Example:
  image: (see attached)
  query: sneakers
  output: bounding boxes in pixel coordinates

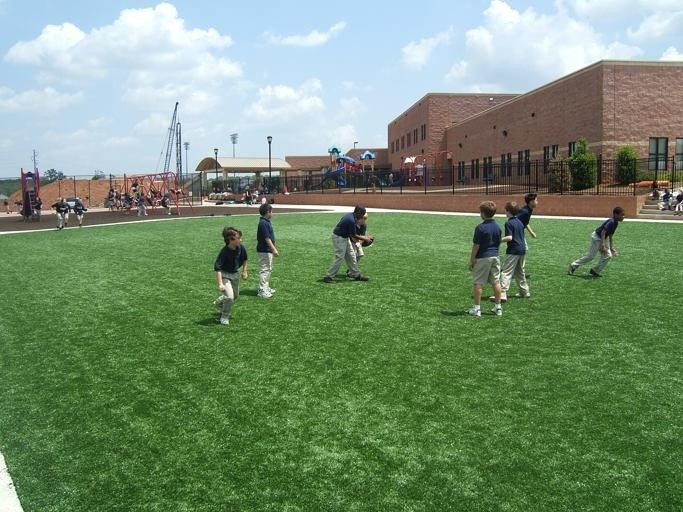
[256,292,273,299]
[567,262,577,275]
[356,274,369,281]
[323,276,334,283]
[220,318,230,325]
[523,273,531,278]
[268,287,276,293]
[213,301,222,308]
[346,269,355,278]
[466,309,481,316]
[589,269,602,278]
[491,308,502,316]
[489,293,507,302]
[515,293,530,298]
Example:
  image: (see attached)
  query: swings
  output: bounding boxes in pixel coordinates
[109,172,182,209]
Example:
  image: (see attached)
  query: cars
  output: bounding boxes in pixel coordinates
[63,198,79,210]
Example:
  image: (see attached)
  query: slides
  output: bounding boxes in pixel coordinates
[19,191,33,216]
[329,166,347,186]
[375,176,389,186]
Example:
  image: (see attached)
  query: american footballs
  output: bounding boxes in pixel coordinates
[362,235,374,246]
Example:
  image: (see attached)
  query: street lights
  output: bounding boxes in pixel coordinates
[229,133,239,159]
[213,148,219,182]
[267,135,273,194]
[183,141,190,180]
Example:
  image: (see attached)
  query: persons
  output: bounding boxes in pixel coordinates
[3,196,87,230]
[651,180,683,216]
[467,201,503,316]
[240,186,259,206]
[488,201,530,303]
[323,202,373,284]
[213,226,248,325]
[110,179,182,216]
[515,194,538,238]
[568,207,625,277]
[256,204,279,298]
[346,211,368,278]
